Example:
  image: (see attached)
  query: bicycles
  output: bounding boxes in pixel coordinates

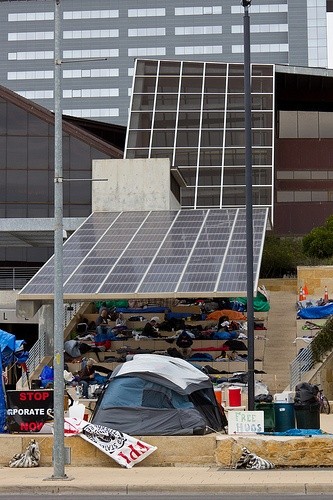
[315,384,331,415]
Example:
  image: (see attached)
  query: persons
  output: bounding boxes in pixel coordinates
[96,310,109,327]
[115,311,127,326]
[76,360,96,399]
[176,330,193,348]
[26,436,48,459]
[142,320,161,338]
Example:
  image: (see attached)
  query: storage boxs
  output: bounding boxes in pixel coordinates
[274,392,294,404]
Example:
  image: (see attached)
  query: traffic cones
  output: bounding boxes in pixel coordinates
[323,285,330,301]
[298,286,304,301]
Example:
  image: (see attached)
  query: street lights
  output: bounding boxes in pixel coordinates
[237,0,255,410]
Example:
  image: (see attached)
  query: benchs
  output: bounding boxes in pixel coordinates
[65,290,270,402]
[295,318,329,358]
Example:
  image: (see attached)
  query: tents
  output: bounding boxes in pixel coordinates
[88,353,228,435]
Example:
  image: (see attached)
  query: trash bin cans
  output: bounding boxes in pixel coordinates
[274,403,295,432]
[293,403,321,429]
[254,402,276,432]
